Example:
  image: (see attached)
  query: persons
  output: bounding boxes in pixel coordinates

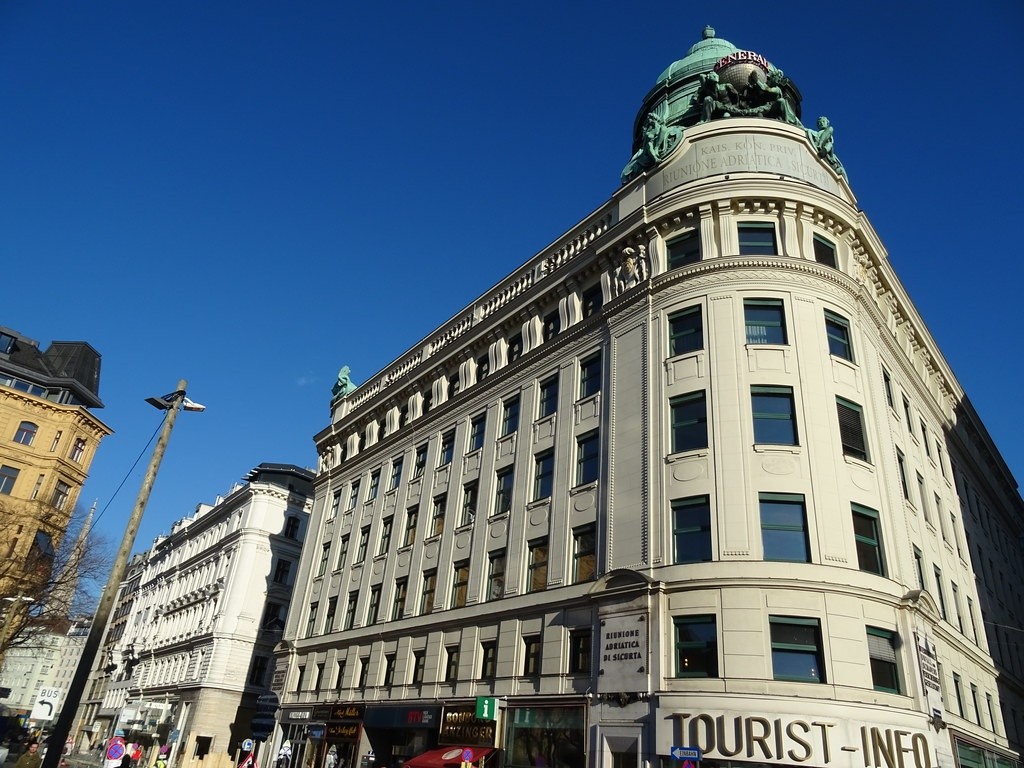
[808,116,834,158]
[113,742,143,768]
[14,741,43,768]
[691,71,739,127]
[750,69,801,124]
[642,112,667,164]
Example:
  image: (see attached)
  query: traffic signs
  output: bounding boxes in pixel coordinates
[671,747,700,761]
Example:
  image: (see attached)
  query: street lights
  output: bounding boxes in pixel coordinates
[39,379,206,768]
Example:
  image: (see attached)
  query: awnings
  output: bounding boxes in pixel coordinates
[398,745,500,768]
